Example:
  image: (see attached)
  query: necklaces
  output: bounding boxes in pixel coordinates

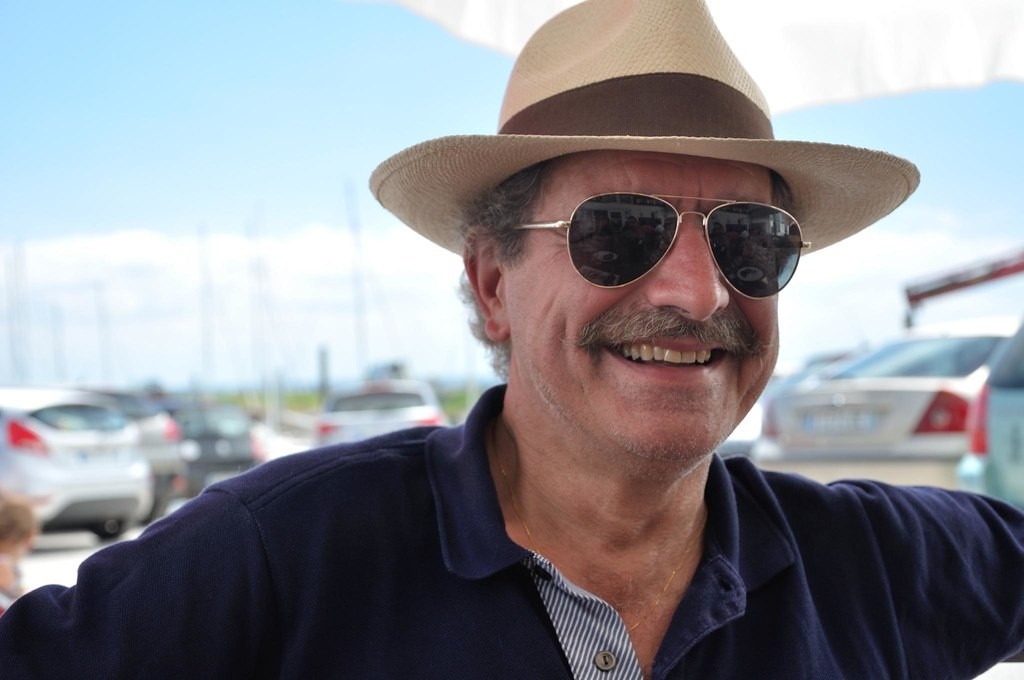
[491,416,708,631]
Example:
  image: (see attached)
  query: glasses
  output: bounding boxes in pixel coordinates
[511,190,812,300]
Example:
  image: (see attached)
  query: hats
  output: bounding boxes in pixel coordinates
[366,0,922,267]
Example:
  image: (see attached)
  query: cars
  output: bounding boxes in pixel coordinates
[749,317,1024,505]
[0,386,154,540]
[163,389,268,499]
[309,377,450,449]
[97,386,186,531]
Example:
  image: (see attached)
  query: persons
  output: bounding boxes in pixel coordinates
[591,208,802,295]
[0,0,1024,680]
[0,495,41,618]
